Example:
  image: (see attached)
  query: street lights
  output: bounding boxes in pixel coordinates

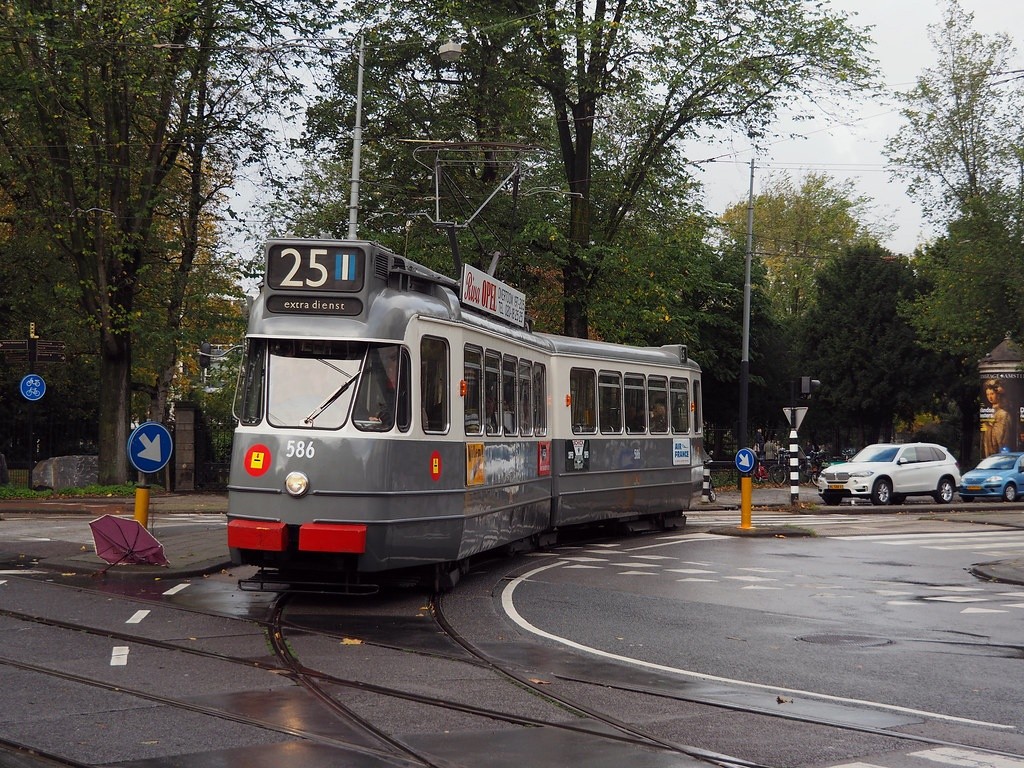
[790,378,821,505]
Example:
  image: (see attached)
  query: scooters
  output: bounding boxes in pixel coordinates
[704,451,717,502]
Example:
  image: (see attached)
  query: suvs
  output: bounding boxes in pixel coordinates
[817,443,963,505]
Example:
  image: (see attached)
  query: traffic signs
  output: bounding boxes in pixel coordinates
[0,339,66,363]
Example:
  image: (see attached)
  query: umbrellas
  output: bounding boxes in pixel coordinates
[88,514,171,574]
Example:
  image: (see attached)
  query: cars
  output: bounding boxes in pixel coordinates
[958,451,1024,503]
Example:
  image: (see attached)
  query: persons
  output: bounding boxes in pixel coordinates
[626,407,644,433]
[650,398,667,432]
[369,405,428,430]
[754,429,829,480]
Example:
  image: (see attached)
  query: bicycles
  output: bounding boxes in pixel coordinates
[749,446,855,487]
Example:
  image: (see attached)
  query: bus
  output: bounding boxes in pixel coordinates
[197,237,704,604]
